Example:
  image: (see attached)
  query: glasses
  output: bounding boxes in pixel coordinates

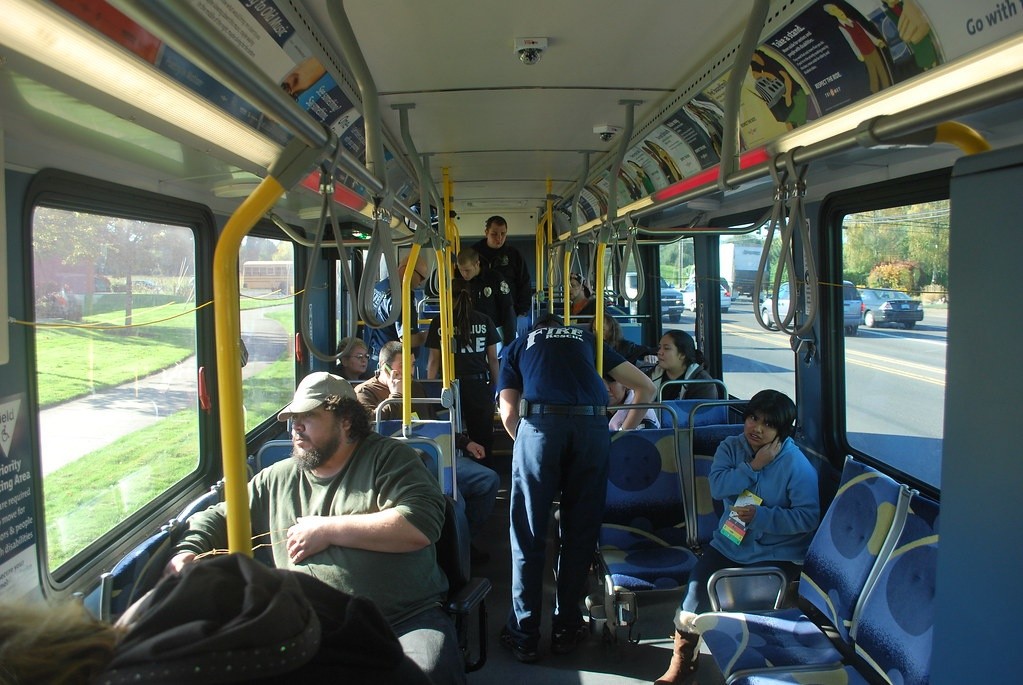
[414,269,426,282]
[344,353,370,360]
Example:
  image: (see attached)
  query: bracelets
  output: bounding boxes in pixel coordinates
[617,424,624,431]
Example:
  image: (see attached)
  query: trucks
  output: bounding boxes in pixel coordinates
[688,242,772,302]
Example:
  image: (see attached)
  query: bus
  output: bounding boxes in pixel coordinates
[242,261,294,295]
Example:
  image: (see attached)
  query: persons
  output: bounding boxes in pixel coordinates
[471,216,532,317]
[423,277,503,468]
[161,371,467,685]
[654,389,821,685]
[452,247,516,348]
[354,341,499,568]
[333,337,373,380]
[570,272,612,324]
[588,313,658,370]
[0,552,427,685]
[363,253,429,379]
[500,320,659,663]
[602,367,661,431]
[643,329,719,399]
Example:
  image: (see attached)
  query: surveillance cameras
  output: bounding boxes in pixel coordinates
[514,37,548,65]
[593,126,622,143]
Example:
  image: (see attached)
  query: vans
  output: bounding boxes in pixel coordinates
[62,273,112,294]
[758,279,864,336]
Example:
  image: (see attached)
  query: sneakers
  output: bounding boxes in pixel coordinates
[550,623,591,653]
[497,623,540,664]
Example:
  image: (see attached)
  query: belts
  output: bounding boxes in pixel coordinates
[528,405,607,416]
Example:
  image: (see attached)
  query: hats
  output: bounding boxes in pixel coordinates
[277,371,358,423]
[570,272,592,299]
[532,314,563,328]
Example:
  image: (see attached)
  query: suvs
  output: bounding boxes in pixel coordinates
[602,271,685,322]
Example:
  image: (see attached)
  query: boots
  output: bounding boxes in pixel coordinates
[653,608,703,685]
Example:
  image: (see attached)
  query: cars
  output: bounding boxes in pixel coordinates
[115,280,156,293]
[685,274,731,294]
[856,288,925,329]
[679,280,731,314]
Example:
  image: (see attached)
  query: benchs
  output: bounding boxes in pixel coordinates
[98,298,940,684]
